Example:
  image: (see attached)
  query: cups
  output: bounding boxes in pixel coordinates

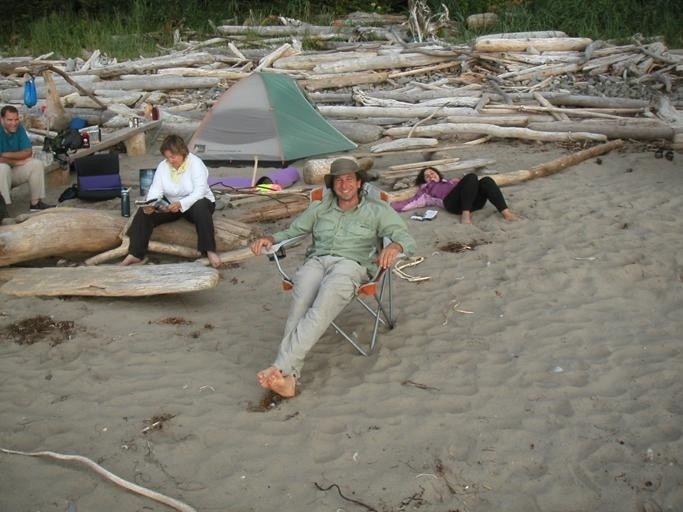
[134,118,138,128]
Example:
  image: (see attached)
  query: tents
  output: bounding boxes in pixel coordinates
[185,70,359,166]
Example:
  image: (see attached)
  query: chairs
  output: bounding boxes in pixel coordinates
[259,180,395,358]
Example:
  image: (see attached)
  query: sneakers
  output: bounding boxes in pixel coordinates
[30,200,55,212]
[25,80,37,107]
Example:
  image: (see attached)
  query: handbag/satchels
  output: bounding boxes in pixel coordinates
[43,127,82,153]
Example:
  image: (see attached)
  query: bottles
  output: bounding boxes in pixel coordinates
[128,114,133,128]
[152,105,158,121]
[121,187,130,217]
[81,132,90,147]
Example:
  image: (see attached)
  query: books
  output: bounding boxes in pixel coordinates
[134,198,172,214]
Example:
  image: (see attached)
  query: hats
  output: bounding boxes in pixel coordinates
[325,158,366,187]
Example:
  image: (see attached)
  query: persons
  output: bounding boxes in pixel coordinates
[391,167,517,224]
[122,135,220,269]
[0,105,57,218]
[251,158,417,399]
